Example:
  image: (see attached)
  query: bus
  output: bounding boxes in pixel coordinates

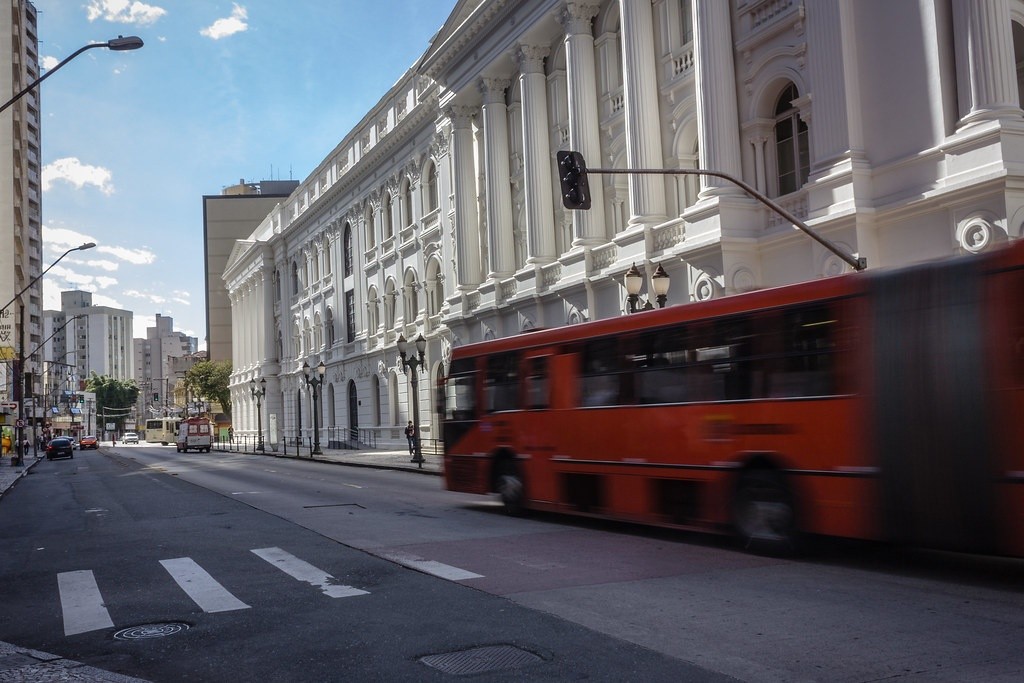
[439,235,1024,579]
[174,417,213,453]
[145,416,184,445]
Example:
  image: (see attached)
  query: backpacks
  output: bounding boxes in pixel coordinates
[406,425,414,436]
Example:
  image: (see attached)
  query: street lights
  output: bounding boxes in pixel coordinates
[623,259,671,364]
[396,332,428,463]
[249,377,267,451]
[14,305,89,466]
[32,348,81,458]
[303,360,326,454]
[153,378,169,417]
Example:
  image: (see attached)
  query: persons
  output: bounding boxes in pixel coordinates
[23,438,31,455]
[227,425,234,442]
[36,436,41,450]
[405,421,415,455]
[14,439,19,453]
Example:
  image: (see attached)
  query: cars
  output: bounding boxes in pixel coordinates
[123,433,139,444]
[79,435,99,450]
[45,436,77,460]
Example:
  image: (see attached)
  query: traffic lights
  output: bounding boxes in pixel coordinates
[80,394,85,404]
[154,392,159,401]
[557,150,594,210]
